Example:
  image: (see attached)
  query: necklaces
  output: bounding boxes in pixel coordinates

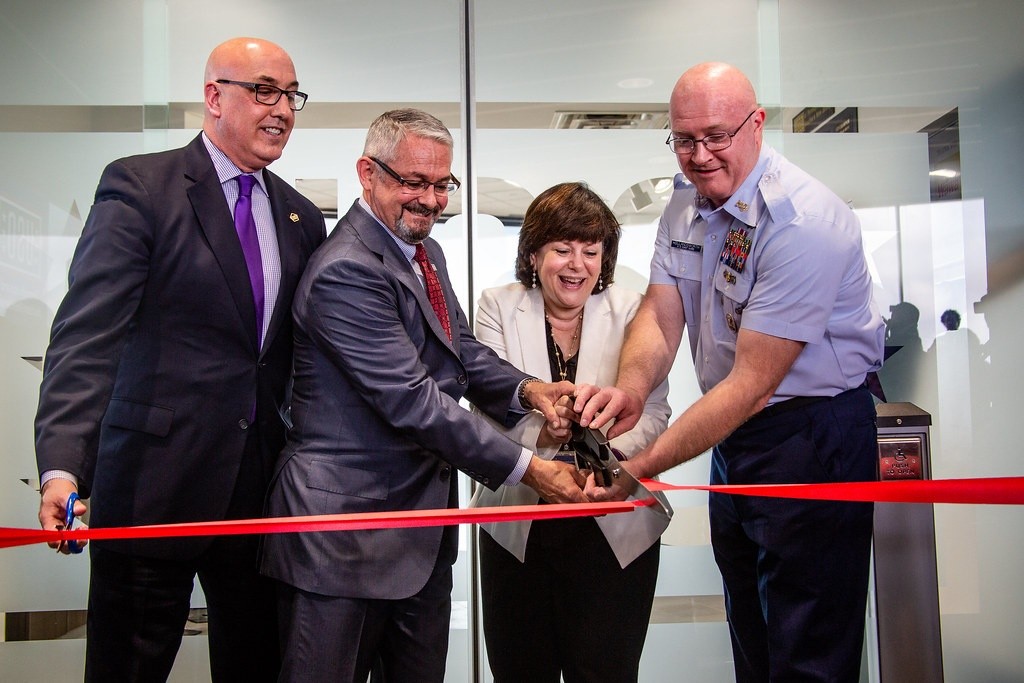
[543,305,582,382]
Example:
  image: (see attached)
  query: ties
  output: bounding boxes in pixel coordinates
[412,242,453,347]
[230,174,265,350]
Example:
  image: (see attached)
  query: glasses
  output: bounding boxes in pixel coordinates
[369,156,461,197]
[216,79,309,111]
[665,110,755,155]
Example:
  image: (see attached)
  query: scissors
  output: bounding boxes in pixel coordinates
[54,491,84,554]
[567,396,671,522]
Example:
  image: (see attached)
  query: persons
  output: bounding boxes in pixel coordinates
[940,310,961,329]
[881,302,922,352]
[465,182,671,683]
[574,63,885,683]
[252,108,603,683]
[34,37,326,683]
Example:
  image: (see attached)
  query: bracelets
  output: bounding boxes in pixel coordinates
[519,378,545,409]
